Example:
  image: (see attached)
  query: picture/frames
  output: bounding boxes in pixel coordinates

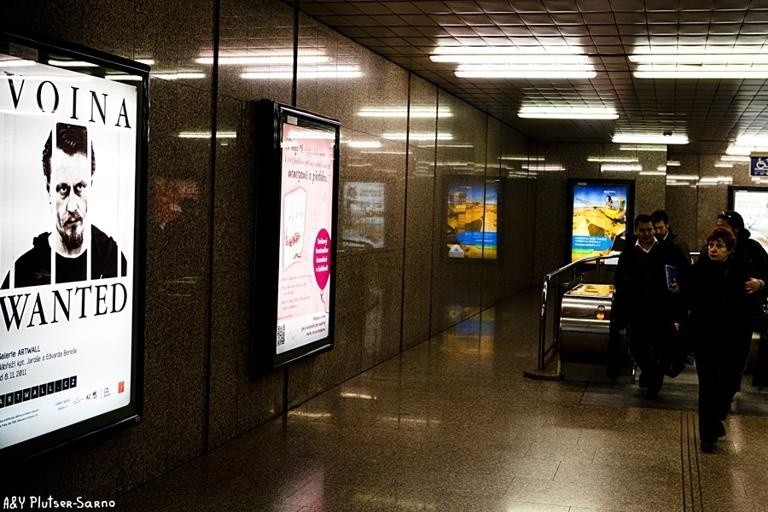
[1,18,157,474]
[437,172,509,270]
[556,176,636,265]
[243,95,342,392]
[340,174,398,267]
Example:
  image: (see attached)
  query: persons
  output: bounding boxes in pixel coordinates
[610,210,767,453]
[1,123,127,289]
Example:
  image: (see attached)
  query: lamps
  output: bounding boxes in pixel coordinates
[421,51,768,146]
